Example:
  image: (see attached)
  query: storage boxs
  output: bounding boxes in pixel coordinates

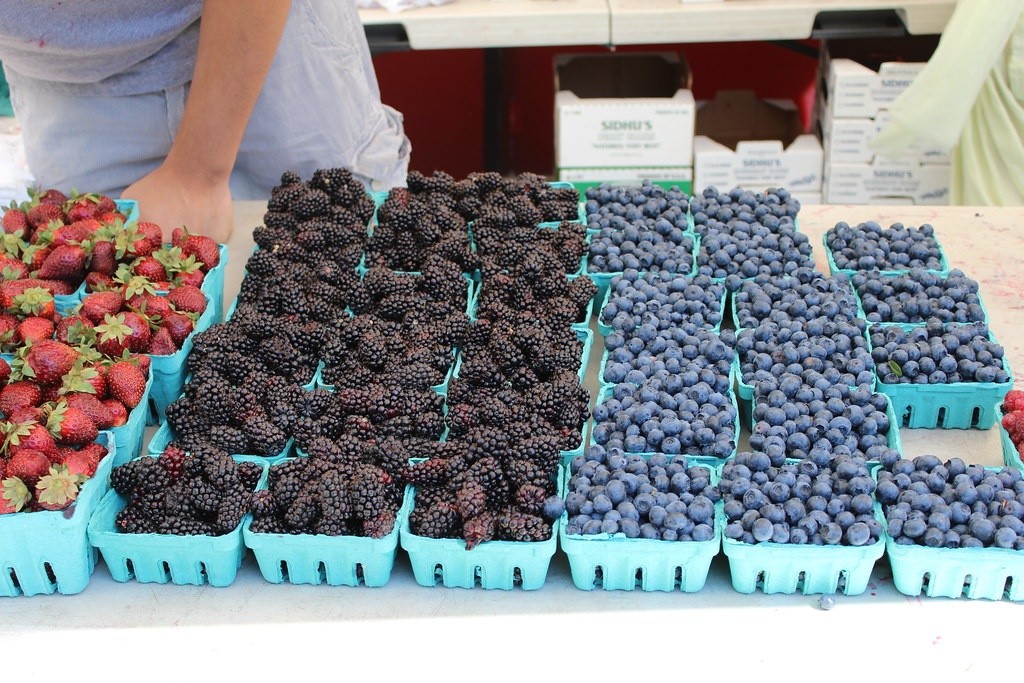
[554,38,966,202]
[0,168,1024,600]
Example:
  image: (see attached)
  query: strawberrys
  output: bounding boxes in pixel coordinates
[1001,389,1024,464]
[0,183,219,515]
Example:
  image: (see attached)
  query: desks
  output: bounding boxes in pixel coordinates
[358,0,960,182]
[0,197,1024,630]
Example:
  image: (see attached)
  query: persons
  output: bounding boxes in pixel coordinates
[0,0,411,245]
